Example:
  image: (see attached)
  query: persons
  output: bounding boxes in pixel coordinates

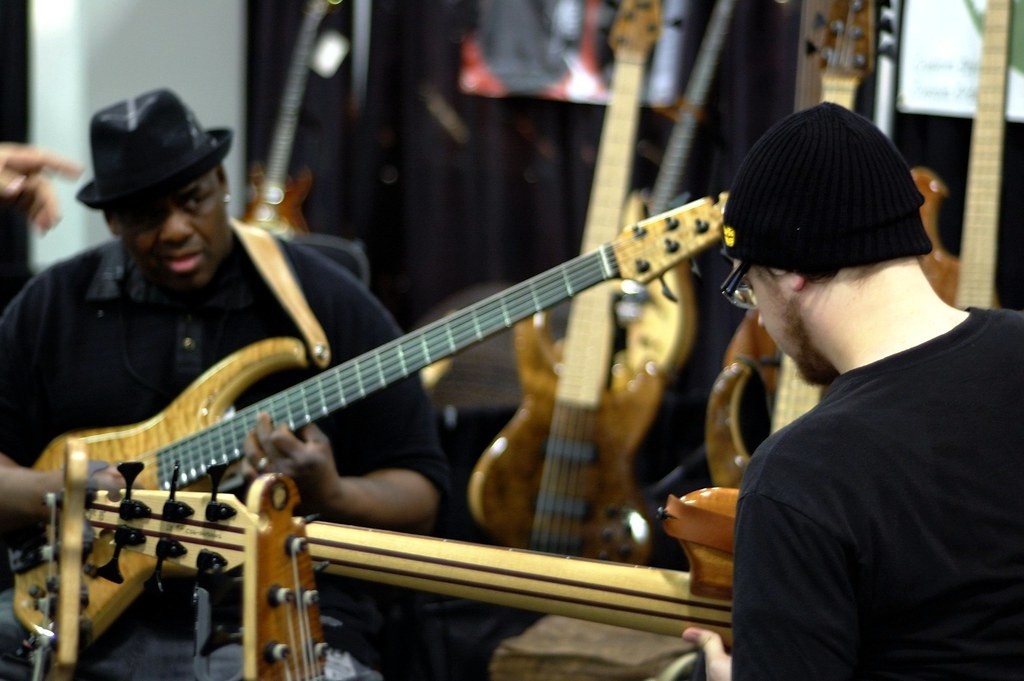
[681,102,1023,681]
[0,86,533,680]
[0,141,83,235]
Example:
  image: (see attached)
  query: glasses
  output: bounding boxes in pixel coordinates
[719,260,756,310]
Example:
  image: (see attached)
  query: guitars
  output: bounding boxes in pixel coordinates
[36,185,732,495]
[704,0,1024,488]
[236,0,342,236]
[466,1,700,568]
[40,437,735,681]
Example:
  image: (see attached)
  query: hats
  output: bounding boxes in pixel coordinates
[720,102,932,273]
[75,89,237,209]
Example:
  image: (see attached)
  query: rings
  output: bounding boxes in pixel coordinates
[256,454,269,470]
